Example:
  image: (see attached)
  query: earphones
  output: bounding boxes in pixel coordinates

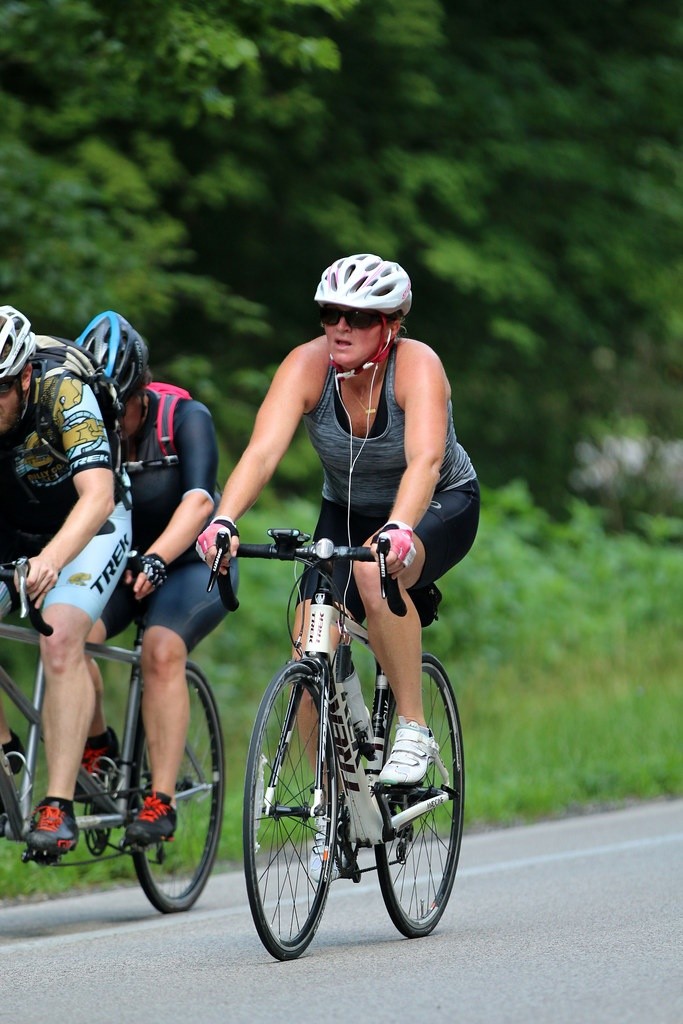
[387,329,392,343]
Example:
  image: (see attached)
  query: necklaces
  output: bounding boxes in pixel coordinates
[344,381,376,414]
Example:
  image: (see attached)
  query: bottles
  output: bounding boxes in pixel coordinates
[342,661,368,733]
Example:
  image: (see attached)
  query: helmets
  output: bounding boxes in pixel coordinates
[0,304,36,380]
[74,310,147,402]
[314,253,412,316]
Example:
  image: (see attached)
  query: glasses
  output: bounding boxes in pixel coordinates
[0,358,30,393]
[318,304,380,329]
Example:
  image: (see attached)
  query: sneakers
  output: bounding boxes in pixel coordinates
[125,789,177,843]
[74,725,121,798]
[27,800,78,853]
[378,716,439,786]
[309,813,355,882]
[2,728,24,775]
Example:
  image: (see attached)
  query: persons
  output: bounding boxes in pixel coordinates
[196,253,481,882]
[76,312,241,845]
[0,304,132,855]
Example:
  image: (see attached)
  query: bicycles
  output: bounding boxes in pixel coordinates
[0,547,228,914]
[209,529,469,963]
